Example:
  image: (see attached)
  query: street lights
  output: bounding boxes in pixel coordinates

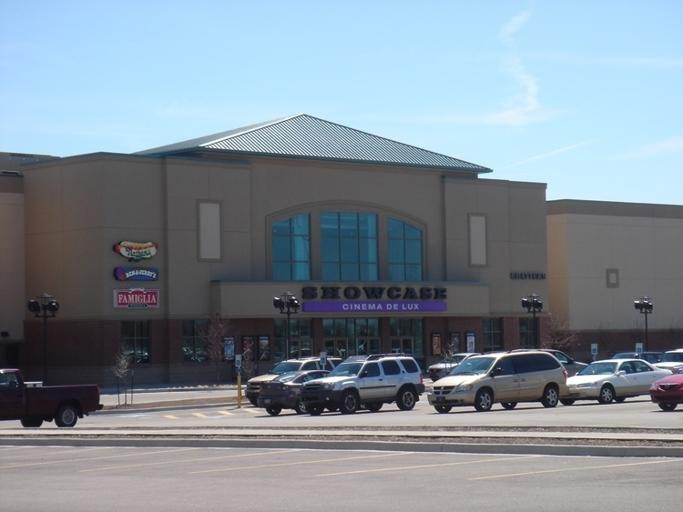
[520,294,544,348]
[273,290,300,360]
[27,292,60,385]
[632,295,653,351]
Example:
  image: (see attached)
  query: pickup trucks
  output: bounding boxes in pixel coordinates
[0,368,104,427]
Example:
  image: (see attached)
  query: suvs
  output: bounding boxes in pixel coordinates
[428,352,490,383]
[299,353,426,416]
[511,348,595,377]
[427,350,570,414]
[244,356,350,407]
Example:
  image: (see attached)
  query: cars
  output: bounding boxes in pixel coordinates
[256,370,336,415]
[558,359,673,406]
[605,348,683,374]
[649,372,683,411]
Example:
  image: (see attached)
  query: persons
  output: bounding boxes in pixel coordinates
[445,343,455,358]
